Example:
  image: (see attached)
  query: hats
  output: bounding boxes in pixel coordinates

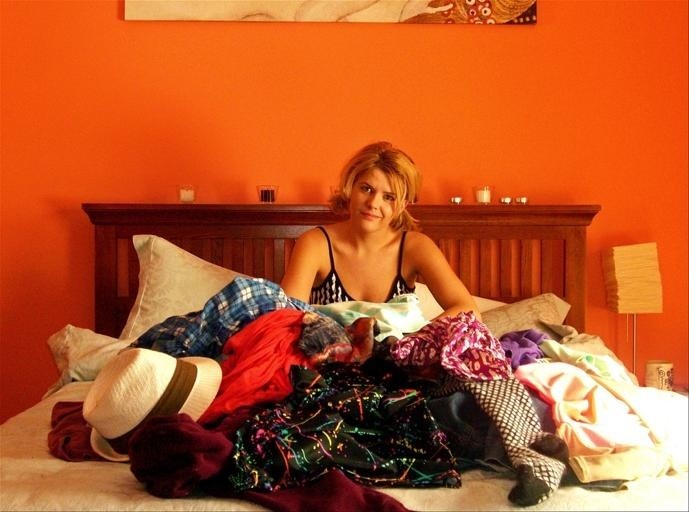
[81,346,223,463]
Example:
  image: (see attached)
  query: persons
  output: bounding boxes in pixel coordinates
[280,140,484,328]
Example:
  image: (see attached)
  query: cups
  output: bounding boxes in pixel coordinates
[176,184,199,203]
[256,185,279,204]
[472,184,495,205]
[644,360,674,392]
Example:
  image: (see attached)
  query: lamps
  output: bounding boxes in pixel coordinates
[600,241,666,375]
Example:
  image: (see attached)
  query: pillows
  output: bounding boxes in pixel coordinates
[48,231,281,391]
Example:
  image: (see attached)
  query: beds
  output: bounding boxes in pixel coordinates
[0,202,687,511]
